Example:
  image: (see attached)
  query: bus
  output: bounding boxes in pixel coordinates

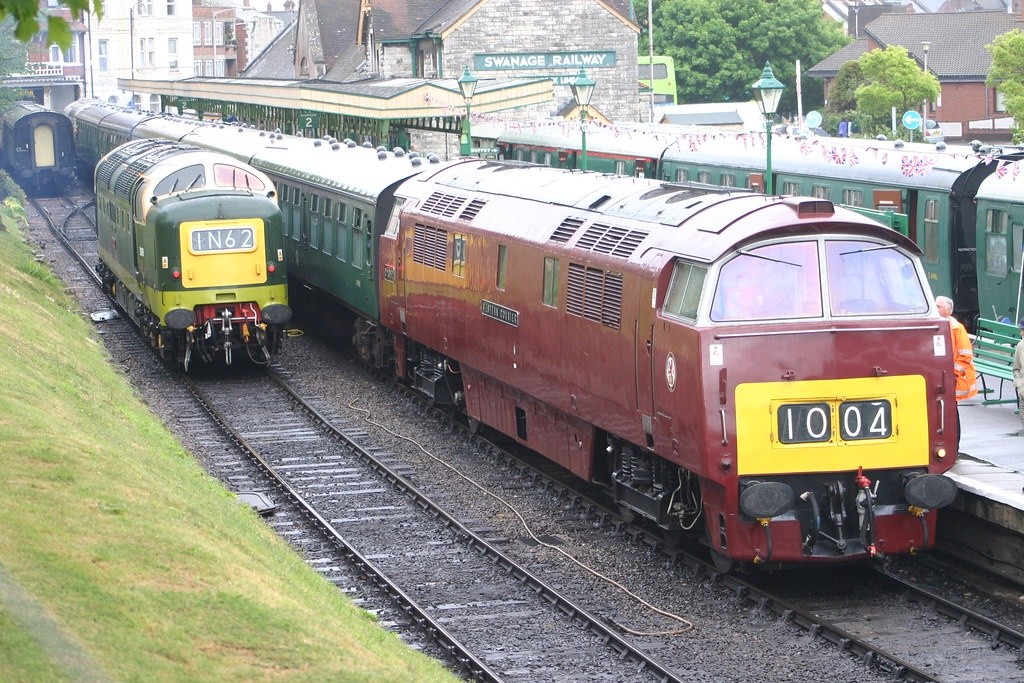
[638,56,679,106]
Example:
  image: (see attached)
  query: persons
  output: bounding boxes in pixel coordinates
[839,118,847,137]
[1013,317,1024,426]
[935,296,977,462]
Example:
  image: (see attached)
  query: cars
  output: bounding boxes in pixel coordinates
[925,119,945,145]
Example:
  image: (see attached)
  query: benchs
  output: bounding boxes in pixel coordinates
[971,318,1023,415]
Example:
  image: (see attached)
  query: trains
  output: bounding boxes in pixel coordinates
[0,98,82,196]
[61,99,961,588]
[91,136,295,368]
[496,115,1023,336]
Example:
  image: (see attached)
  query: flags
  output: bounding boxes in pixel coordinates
[424,94,1021,180]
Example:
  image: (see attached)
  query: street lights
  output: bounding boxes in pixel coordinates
[750,60,786,195]
[920,40,932,138]
[567,66,595,171]
[457,66,478,161]
